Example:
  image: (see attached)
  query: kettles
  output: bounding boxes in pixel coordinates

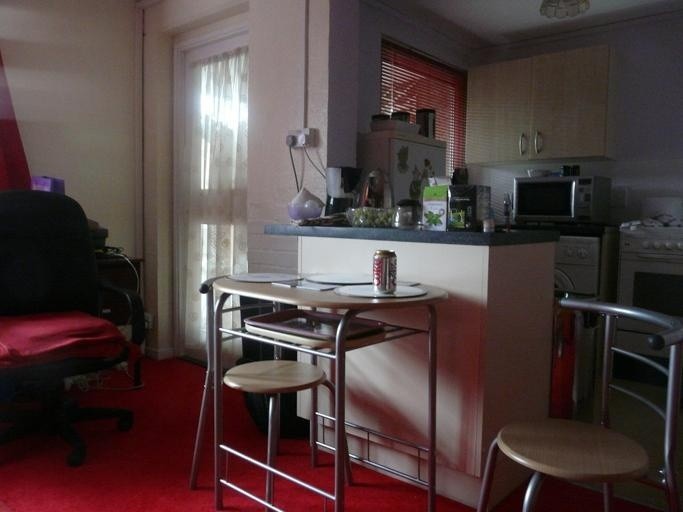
[392,198,421,231]
[353,164,391,208]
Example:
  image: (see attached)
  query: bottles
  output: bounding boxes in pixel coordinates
[559,166,579,175]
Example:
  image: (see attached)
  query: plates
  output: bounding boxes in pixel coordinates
[225,269,302,284]
[306,272,378,284]
[334,283,428,299]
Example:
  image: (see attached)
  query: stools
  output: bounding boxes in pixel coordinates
[217,355,355,512]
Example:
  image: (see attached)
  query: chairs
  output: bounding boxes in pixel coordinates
[1,190,144,469]
[475,296,683,511]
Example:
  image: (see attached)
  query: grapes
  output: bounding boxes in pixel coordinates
[352,207,395,226]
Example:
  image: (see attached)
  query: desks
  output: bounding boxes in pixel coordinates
[209,273,449,511]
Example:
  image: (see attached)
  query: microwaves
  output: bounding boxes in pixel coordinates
[512,176,595,223]
[448,184,481,225]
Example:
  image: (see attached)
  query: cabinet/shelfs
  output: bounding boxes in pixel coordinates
[463,45,623,169]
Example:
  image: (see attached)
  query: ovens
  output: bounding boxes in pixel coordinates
[618,252,683,333]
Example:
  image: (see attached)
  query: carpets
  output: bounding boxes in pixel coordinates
[1,357,661,512]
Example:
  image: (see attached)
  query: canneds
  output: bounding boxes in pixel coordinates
[374,250,397,294]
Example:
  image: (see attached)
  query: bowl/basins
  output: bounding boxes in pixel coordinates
[343,206,391,229]
[526,167,550,177]
[370,120,420,137]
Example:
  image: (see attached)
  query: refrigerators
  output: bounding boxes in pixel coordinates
[354,133,447,216]
